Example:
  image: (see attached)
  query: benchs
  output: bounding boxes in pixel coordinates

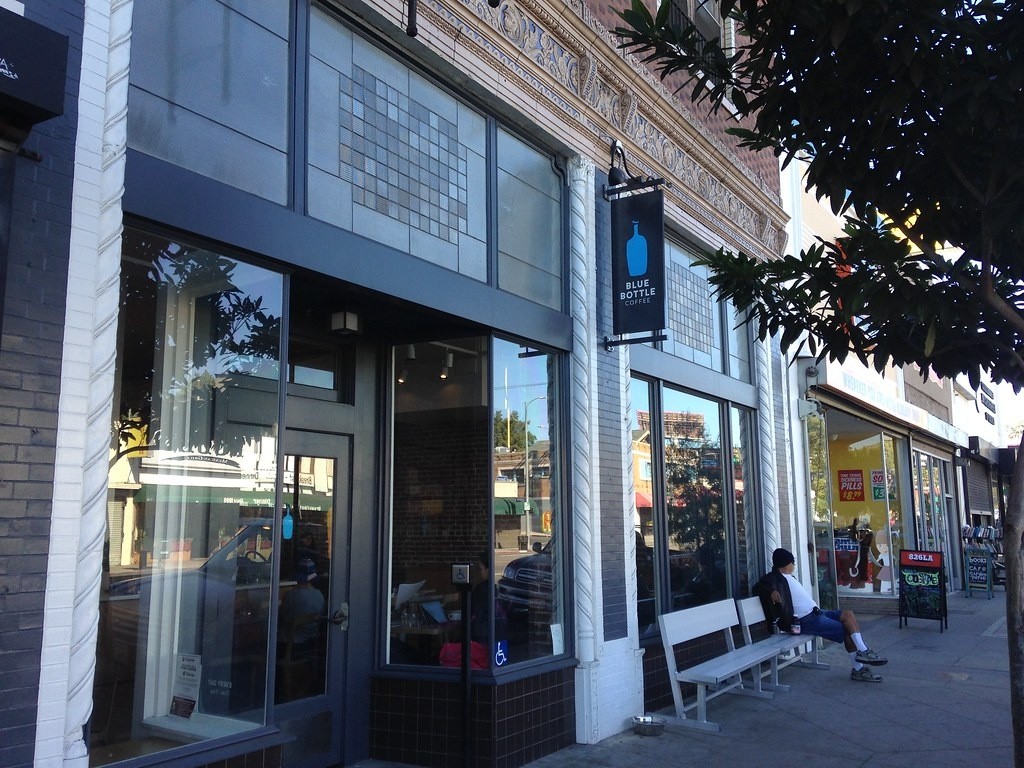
[728,595,830,693]
[645,599,781,730]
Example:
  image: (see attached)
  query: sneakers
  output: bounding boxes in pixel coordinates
[855,648,889,666]
[851,665,884,683]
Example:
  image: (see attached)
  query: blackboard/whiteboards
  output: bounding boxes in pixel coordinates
[966,548,993,589]
[898,549,948,620]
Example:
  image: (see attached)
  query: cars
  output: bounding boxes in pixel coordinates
[104,521,333,669]
[499,532,646,625]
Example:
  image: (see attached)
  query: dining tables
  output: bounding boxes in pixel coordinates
[390,600,462,665]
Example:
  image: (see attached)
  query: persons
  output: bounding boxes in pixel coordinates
[299,530,317,551]
[749,546,888,683]
[464,547,532,649]
[682,547,729,608]
[259,559,329,647]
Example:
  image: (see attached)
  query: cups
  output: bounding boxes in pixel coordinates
[448,611,462,622]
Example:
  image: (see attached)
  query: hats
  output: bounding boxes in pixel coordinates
[772,548,794,569]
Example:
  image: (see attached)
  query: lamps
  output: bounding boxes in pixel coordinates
[608,145,641,186]
[397,369,409,384]
[434,352,455,387]
[330,311,359,337]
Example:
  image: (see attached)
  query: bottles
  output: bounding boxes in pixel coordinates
[791,614,801,636]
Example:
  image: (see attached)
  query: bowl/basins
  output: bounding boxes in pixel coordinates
[632,716,667,736]
